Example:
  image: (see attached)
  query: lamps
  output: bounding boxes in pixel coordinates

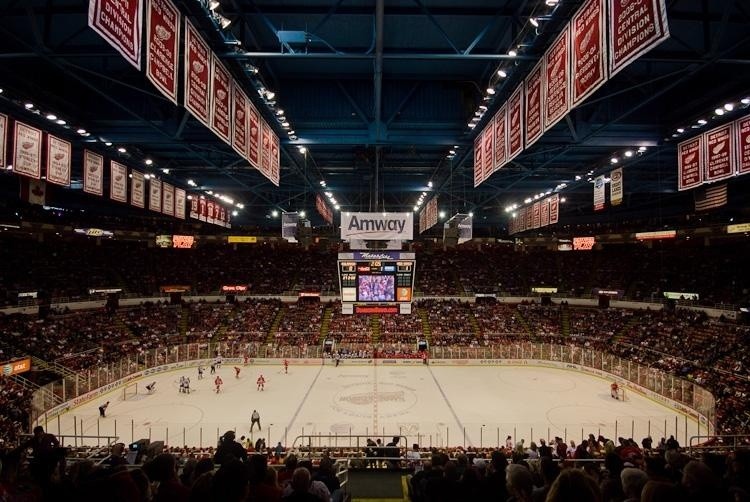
[206,0,232,30]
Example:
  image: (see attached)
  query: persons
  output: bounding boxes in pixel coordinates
[0,241,750,502]
